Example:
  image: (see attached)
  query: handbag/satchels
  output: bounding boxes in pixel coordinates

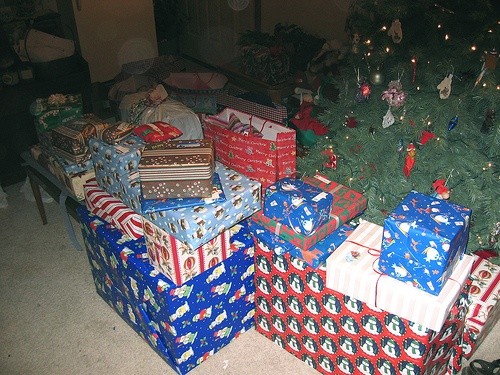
[119,89,204,141]
[190,106,298,200]
[21,17,77,63]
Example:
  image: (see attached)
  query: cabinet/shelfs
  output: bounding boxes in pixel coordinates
[0,52,92,189]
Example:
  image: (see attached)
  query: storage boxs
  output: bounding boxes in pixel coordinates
[28,43,500,375]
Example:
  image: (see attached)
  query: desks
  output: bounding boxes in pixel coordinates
[235,68,295,104]
[27,164,79,225]
